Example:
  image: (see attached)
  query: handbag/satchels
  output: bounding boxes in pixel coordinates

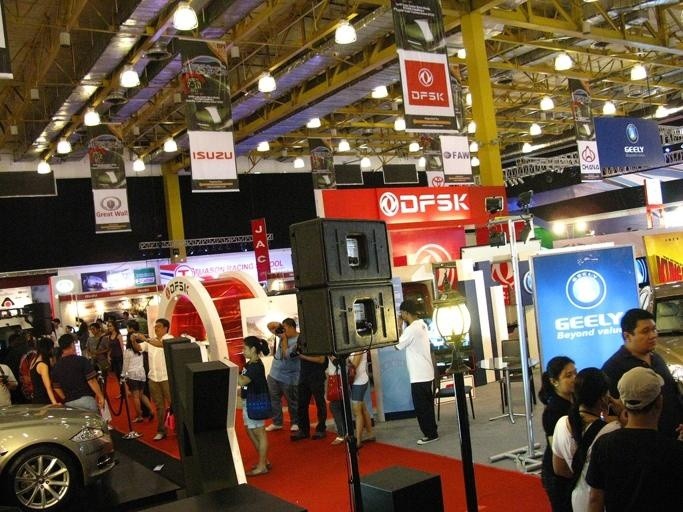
[327,375,342,401]
[246,391,273,419]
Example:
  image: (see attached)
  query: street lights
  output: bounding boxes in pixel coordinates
[431,275,479,507]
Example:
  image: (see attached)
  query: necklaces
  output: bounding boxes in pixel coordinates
[578,410,601,418]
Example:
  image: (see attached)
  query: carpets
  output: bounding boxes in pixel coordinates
[99,368,552,512]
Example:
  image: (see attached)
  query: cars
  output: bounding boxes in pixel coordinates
[0,403,119,507]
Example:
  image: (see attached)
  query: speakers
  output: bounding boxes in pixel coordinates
[288,218,392,289]
[358,464,445,511]
[295,284,398,356]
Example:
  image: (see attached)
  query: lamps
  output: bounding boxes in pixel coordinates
[486,190,535,249]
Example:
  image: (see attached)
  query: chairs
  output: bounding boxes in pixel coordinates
[430,353,475,421]
[498,339,536,416]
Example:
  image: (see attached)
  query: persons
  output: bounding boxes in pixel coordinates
[552,362,627,512]
[535,354,580,512]
[585,366,683,512]
[598,308,683,441]
[0,297,381,483]
[393,300,440,444]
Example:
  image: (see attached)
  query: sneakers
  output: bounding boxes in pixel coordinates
[265,424,283,431]
[290,432,310,438]
[153,433,167,440]
[132,417,144,423]
[417,435,439,444]
[290,424,299,431]
[348,367,356,383]
[312,430,326,439]
[361,436,376,441]
[332,434,348,445]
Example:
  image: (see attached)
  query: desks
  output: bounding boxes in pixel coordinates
[473,354,540,423]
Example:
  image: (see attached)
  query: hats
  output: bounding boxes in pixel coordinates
[400,300,421,316]
[616,367,665,410]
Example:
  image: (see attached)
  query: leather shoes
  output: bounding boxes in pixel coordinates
[246,468,267,476]
[252,463,273,469]
